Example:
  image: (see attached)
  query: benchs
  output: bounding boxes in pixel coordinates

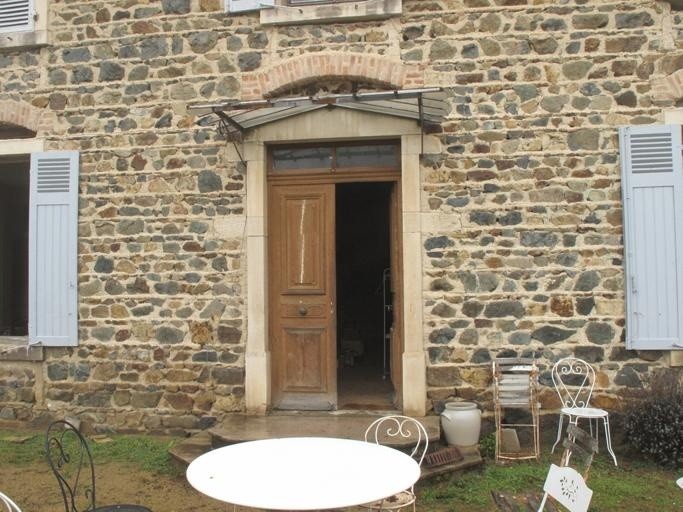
[490,422,599,512]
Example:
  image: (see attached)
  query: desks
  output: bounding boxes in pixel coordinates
[185,437,421,512]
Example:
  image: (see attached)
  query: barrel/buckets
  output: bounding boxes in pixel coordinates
[441,402,482,447]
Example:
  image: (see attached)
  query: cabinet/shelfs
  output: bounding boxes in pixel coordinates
[381,264,392,380]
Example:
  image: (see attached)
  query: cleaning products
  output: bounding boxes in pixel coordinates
[344,344,355,368]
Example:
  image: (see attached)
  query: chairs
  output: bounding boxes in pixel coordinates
[537,463,594,512]
[551,357,618,467]
[358,416,429,512]
[45,419,153,512]
[491,357,541,465]
